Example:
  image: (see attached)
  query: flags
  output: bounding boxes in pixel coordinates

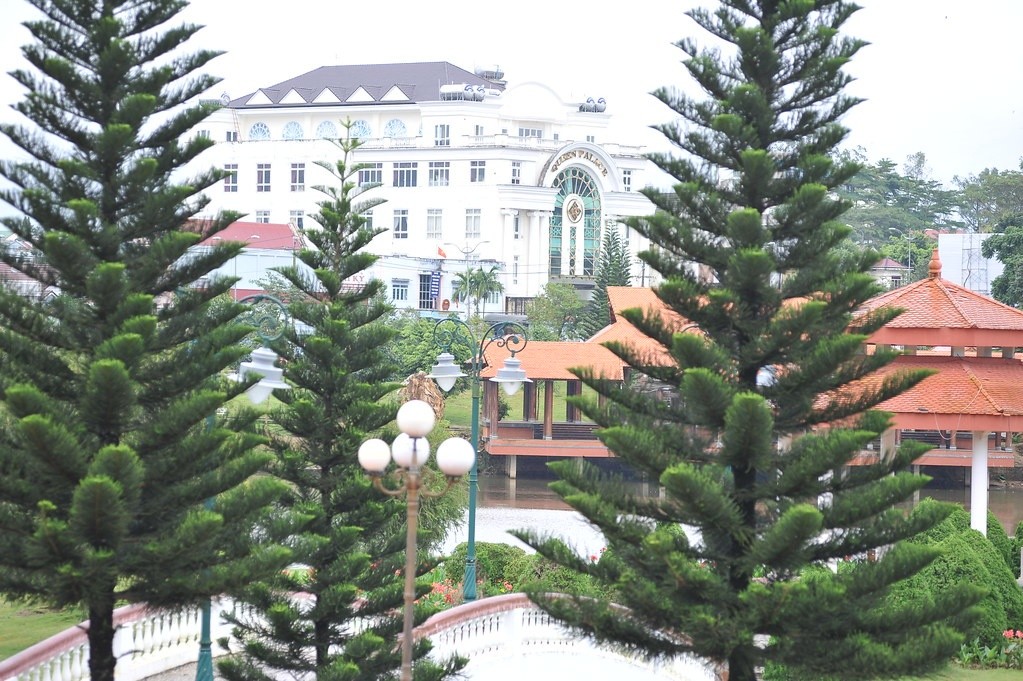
[438,248,446,258]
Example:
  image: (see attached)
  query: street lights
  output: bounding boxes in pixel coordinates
[357,398,476,681]
[182,294,290,681]
[423,316,533,598]
